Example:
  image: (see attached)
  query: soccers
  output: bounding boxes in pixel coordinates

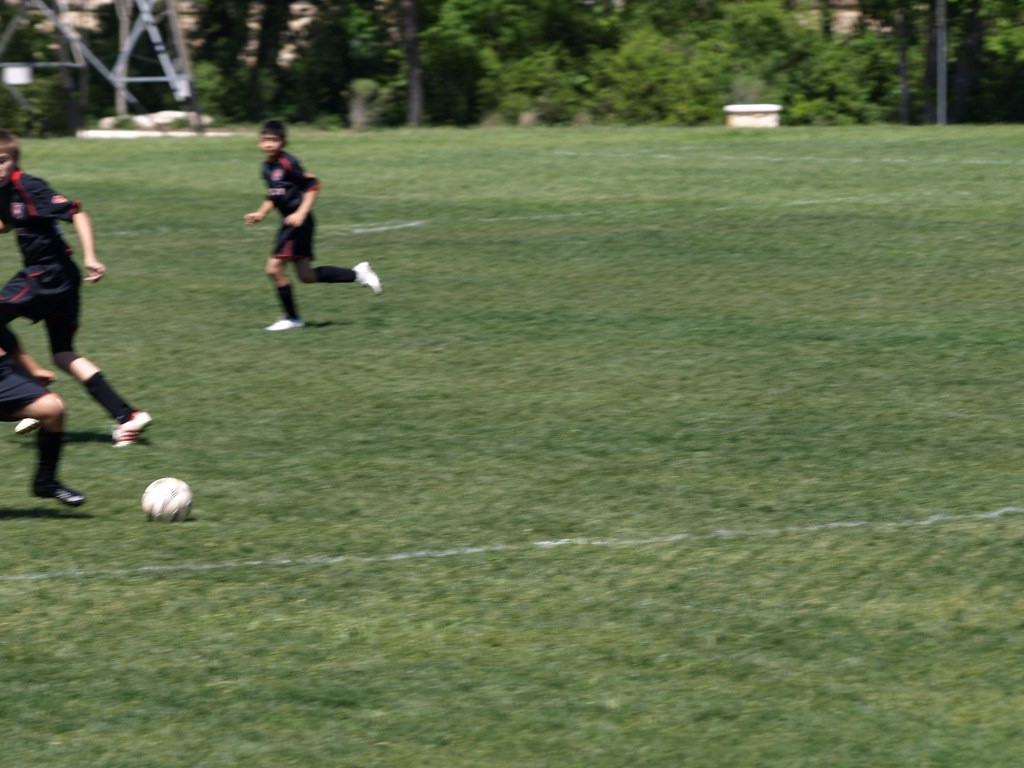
[138,474,196,526]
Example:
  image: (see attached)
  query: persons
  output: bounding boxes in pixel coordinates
[243,120,382,331]
[0,131,152,449]
[0,318,86,507]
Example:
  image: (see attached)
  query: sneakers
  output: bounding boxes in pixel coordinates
[352,262,382,295]
[264,317,304,331]
[13,417,40,434]
[50,485,85,506]
[112,411,152,447]
